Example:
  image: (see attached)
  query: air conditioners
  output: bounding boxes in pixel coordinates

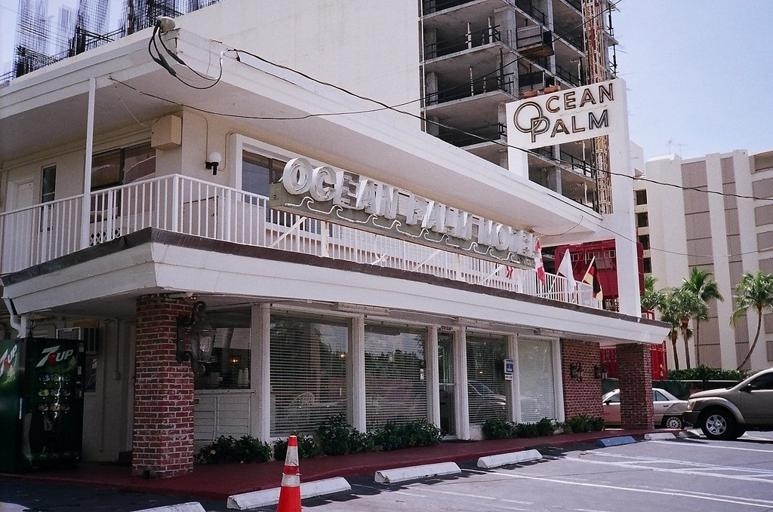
[56,326,97,355]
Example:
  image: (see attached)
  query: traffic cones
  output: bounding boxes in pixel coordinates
[275,435,301,512]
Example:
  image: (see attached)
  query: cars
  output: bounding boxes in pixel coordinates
[467,379,506,424]
[686,368,772,440]
[601,388,688,431]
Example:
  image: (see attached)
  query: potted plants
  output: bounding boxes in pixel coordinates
[590,417,605,431]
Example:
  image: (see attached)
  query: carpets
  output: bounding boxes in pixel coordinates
[441,440,480,443]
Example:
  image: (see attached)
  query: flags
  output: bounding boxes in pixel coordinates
[558,248,576,292]
[582,258,603,302]
[530,238,546,285]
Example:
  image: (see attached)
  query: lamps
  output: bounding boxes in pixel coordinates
[570,360,584,383]
[206,151,222,175]
[172,301,218,377]
[594,361,609,380]
